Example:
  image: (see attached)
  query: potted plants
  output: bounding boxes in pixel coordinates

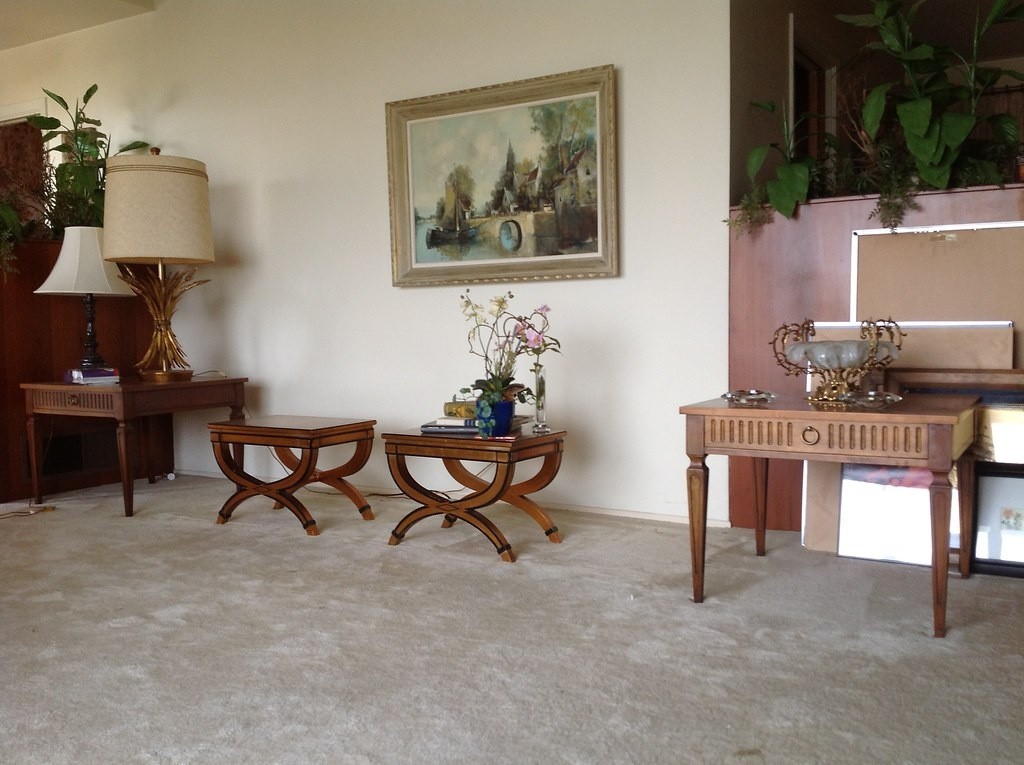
[24,77,158,237]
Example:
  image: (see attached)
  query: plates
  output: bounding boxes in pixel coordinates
[836,391,903,411]
[721,389,776,406]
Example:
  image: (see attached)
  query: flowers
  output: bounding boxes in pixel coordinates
[454,291,560,403]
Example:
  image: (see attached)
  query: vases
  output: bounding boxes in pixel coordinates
[475,401,514,435]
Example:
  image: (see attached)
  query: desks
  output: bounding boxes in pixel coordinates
[681,395,985,639]
[21,375,251,518]
[207,414,377,535]
[381,427,566,563]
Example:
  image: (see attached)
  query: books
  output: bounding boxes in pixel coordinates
[420,400,535,443]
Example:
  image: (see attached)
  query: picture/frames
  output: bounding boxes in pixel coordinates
[969,462,1023,577]
[385,61,616,283]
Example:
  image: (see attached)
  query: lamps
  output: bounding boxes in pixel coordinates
[101,154,218,381]
[33,226,141,368]
